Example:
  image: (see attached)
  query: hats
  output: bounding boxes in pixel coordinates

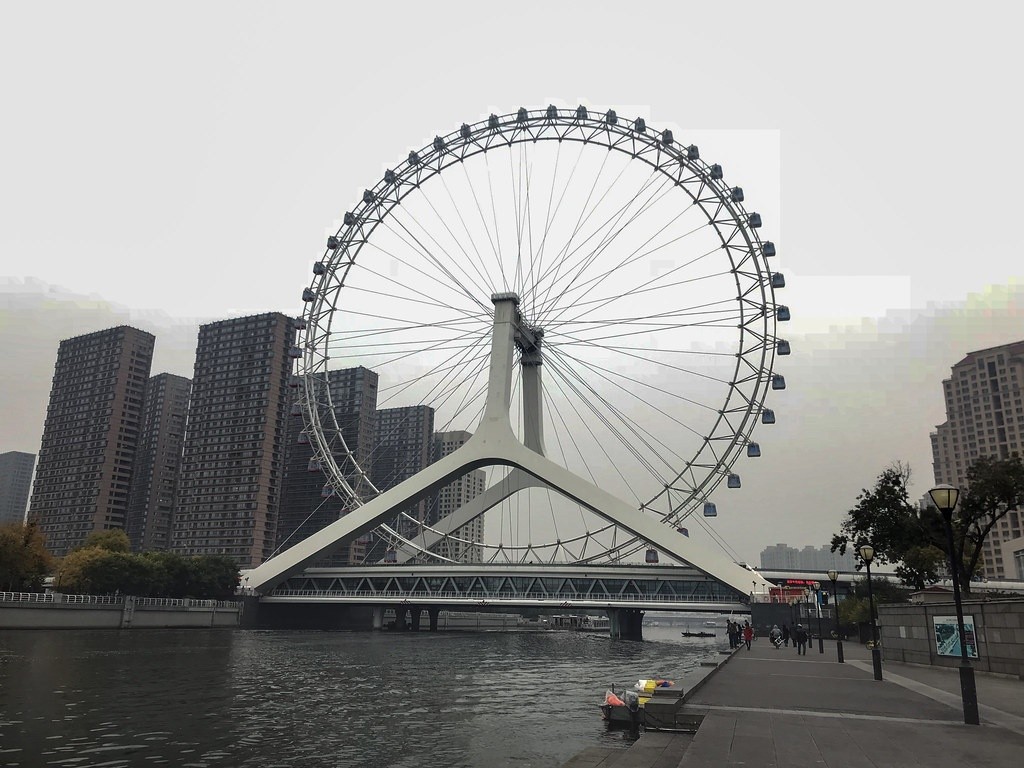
[797,624,802,626]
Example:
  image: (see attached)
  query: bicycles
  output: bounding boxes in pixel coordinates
[865,638,881,650]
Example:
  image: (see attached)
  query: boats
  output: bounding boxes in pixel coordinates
[681,625,717,637]
[599,677,673,721]
[543,612,610,630]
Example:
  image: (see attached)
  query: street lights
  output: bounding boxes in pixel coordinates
[827,568,845,664]
[927,482,983,723]
[804,588,812,647]
[788,598,802,630]
[812,580,824,654]
[859,544,884,681]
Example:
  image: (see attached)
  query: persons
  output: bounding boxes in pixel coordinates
[743,623,754,651]
[789,622,797,648]
[733,621,742,645]
[781,625,790,647]
[770,625,782,645]
[744,620,749,626]
[796,623,808,655]
[726,620,739,649]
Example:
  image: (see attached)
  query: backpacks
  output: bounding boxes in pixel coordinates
[800,632,807,643]
